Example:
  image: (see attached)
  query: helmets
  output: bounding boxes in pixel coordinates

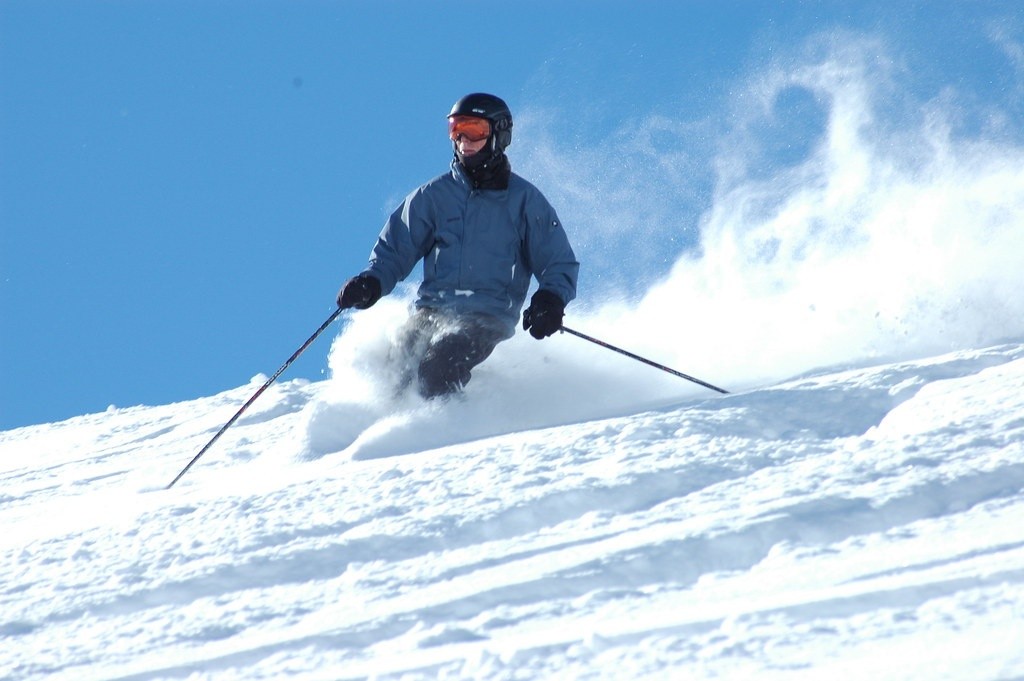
[446,93,513,178]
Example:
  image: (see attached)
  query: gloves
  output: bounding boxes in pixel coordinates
[523,290,565,340]
[336,275,381,310]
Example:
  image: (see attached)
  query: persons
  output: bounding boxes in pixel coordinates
[332,92,580,398]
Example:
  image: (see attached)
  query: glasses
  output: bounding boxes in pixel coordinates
[448,115,492,142]
